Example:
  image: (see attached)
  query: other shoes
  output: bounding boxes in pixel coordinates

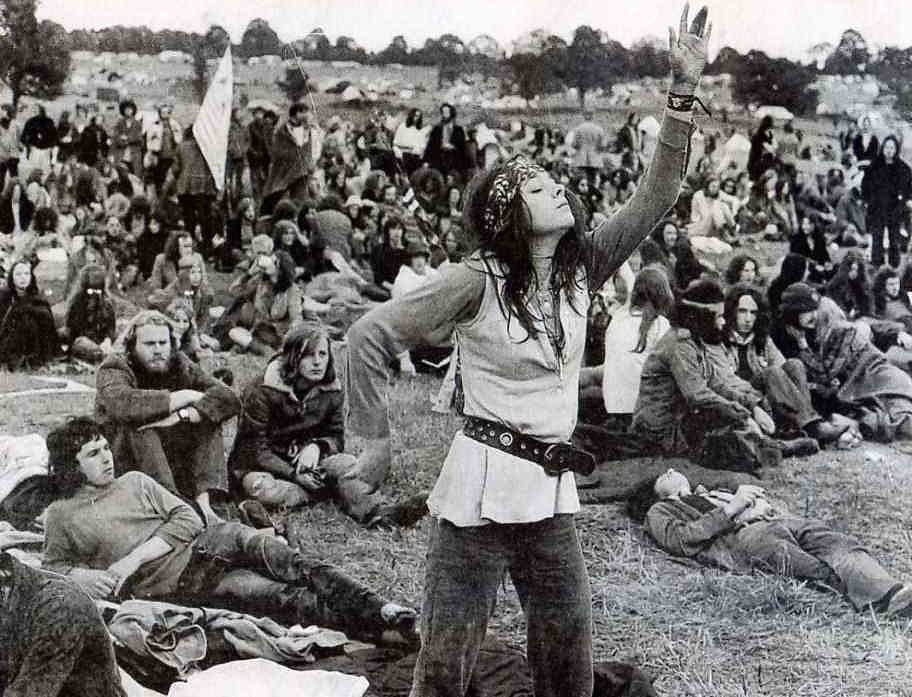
[815,422,850,448]
[765,437,817,455]
[368,489,431,530]
[883,582,912,619]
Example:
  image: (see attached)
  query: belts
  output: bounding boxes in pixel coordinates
[737,516,762,531]
[462,415,595,477]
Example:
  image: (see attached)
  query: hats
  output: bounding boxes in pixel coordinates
[779,282,818,311]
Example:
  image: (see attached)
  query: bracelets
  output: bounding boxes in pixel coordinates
[667,93,694,111]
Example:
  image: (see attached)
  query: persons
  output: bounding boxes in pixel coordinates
[625,468,912,620]
[348,1,713,696]
[0,103,912,472]
[40,418,423,644]
[0,553,127,697]
[690,176,741,246]
[226,325,431,529]
[93,310,242,522]
[860,134,912,267]
[57,262,116,361]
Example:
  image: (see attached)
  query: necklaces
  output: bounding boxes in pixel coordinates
[539,298,563,380]
